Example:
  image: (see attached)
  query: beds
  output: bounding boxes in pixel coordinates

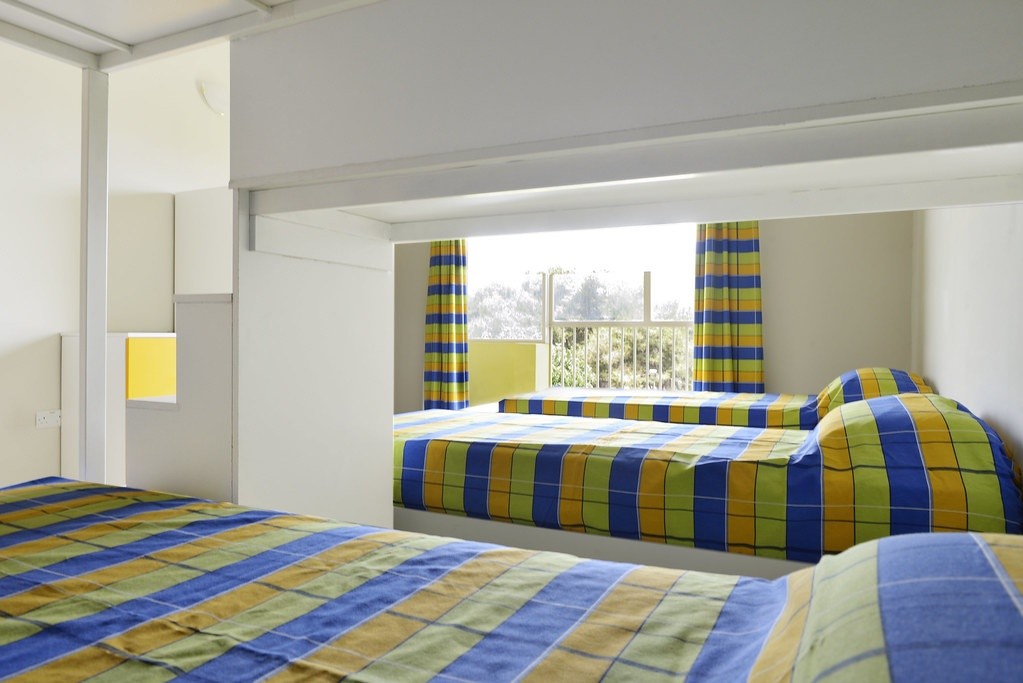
[500,369,936,431]
[394,393,1023,566]
[0,400,1023,683]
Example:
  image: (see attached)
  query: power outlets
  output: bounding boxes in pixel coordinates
[36,409,61,430]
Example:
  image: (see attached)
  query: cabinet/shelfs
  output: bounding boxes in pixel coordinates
[465,337,549,408]
[106,332,177,399]
